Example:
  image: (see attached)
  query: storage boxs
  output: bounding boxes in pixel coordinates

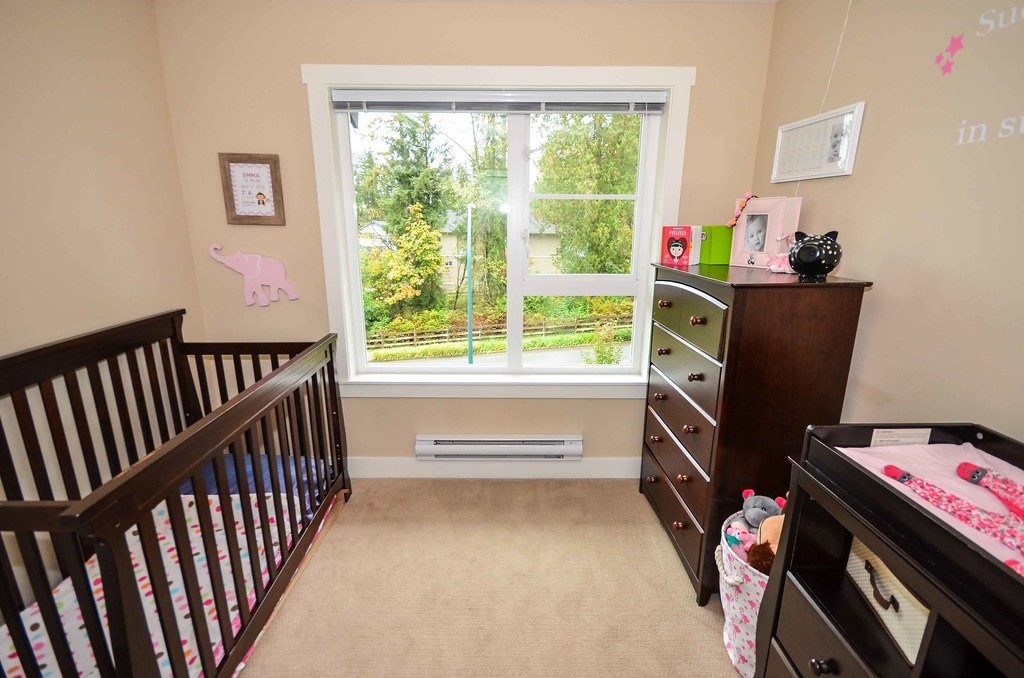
[699,225,731,265]
[660,225,701,266]
[729,195,803,268]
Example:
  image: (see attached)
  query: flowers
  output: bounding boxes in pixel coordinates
[726,191,759,229]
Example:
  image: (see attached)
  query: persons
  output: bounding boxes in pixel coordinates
[744,215,766,252]
[825,123,847,162]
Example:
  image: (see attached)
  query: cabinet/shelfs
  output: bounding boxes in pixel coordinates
[755,422,1024,678]
[638,264,874,606]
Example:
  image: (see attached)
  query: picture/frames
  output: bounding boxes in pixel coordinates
[218,152,286,226]
[770,101,866,184]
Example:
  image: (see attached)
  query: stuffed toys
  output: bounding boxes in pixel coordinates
[723,489,787,576]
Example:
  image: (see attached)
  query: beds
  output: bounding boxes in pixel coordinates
[0,306,352,678]
